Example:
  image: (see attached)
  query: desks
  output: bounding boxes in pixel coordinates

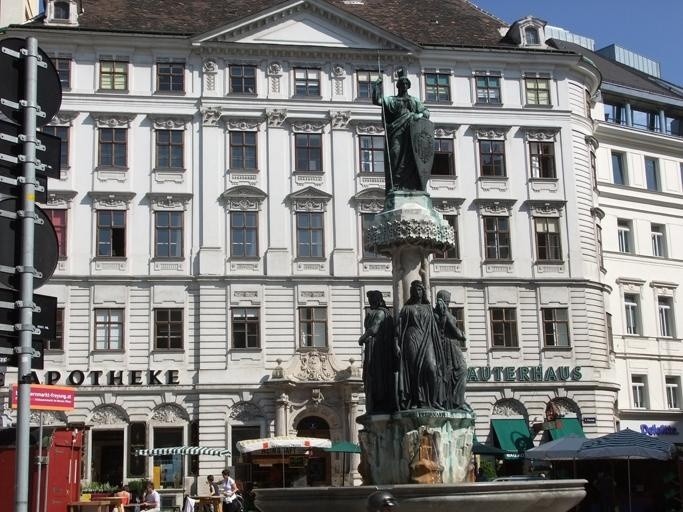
[189,495,226,512]
[67,496,144,512]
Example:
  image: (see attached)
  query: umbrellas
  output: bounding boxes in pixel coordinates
[523,433,595,479]
[576,427,676,511]
[322,439,361,486]
[235,435,332,486]
[467,438,514,480]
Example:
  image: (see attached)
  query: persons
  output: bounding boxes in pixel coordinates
[138,482,160,511]
[370,73,430,189]
[206,474,221,511]
[214,469,238,511]
[430,289,474,414]
[391,279,447,412]
[115,483,132,506]
[357,290,402,412]
